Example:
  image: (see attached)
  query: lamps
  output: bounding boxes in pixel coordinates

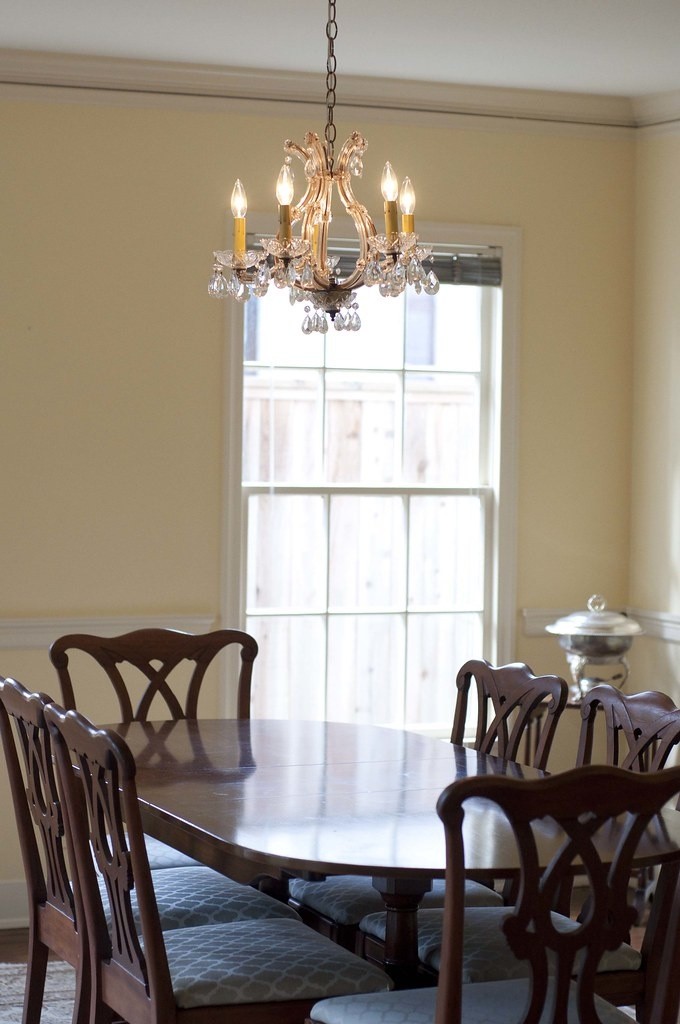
[208,0,440,334]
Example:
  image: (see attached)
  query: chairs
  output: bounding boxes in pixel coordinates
[0,626,680,1024]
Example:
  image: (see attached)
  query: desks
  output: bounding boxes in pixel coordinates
[72,719,680,990]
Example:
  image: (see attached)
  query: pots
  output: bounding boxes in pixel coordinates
[545,595,646,656]
